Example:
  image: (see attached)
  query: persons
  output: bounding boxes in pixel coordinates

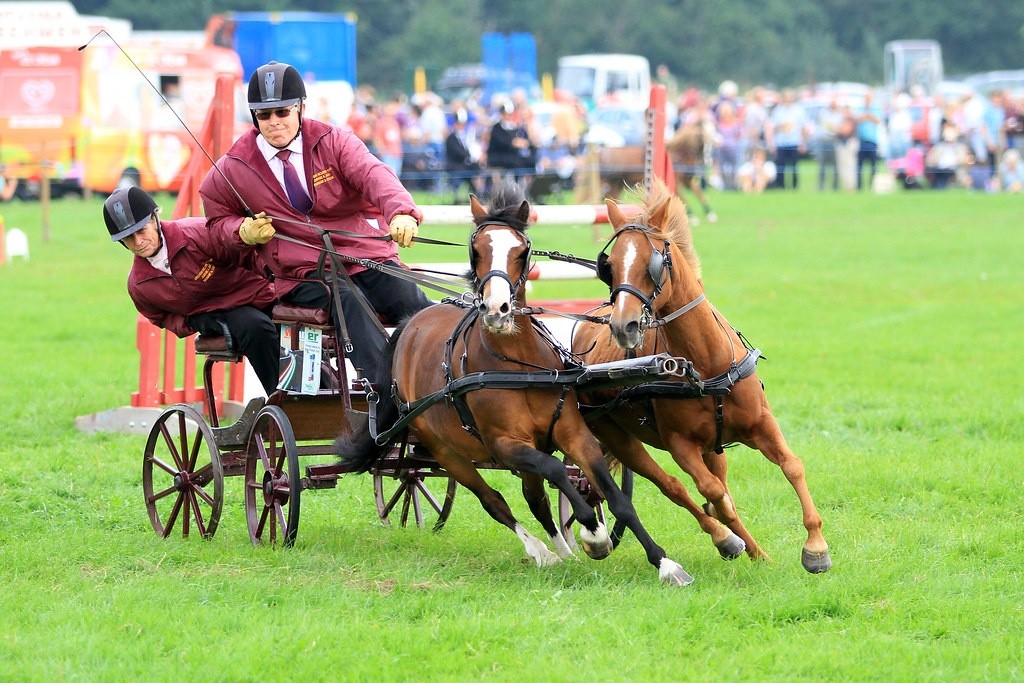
[349,79,1024,204]
[198,60,440,379]
[102,187,279,400]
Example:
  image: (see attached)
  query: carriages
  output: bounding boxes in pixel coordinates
[133,173,833,589]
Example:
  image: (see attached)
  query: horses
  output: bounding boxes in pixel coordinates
[572,172,834,574]
[332,175,695,589]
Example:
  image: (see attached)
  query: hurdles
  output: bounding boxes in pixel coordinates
[71,72,677,439]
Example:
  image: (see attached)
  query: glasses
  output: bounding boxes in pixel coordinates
[255,101,299,120]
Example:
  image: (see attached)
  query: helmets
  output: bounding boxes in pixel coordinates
[248,61,306,128]
[103,186,157,241]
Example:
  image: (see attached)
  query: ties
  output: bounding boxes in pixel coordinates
[274,149,313,214]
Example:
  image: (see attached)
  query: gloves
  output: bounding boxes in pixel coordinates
[263,264,275,282]
[239,212,276,245]
[384,216,419,248]
[187,313,226,338]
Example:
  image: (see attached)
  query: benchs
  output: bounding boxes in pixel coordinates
[183,312,244,422]
[260,254,354,407]
